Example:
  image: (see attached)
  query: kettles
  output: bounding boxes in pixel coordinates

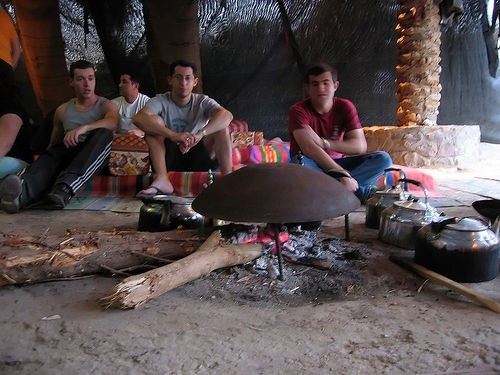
[414,216,500,283]
[138,195,204,233]
[379,178,446,248]
[366,167,406,228]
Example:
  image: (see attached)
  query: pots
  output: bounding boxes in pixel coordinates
[191,162,361,223]
[472,200,500,220]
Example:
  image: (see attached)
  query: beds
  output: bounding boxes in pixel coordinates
[75,120,433,193]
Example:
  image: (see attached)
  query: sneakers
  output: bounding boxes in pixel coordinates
[45,184,75,209]
[0,173,23,214]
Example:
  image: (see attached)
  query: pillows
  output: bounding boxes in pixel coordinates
[229,131,263,147]
[232,136,283,165]
[246,143,292,165]
[228,120,248,133]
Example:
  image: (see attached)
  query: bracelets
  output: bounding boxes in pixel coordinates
[321,138,330,149]
[197,129,206,136]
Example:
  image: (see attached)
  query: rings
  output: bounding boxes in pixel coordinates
[186,137,189,141]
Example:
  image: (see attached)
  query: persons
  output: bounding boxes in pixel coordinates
[0,60,122,215]
[110,70,151,130]
[0,59,52,180]
[131,61,234,198]
[287,64,393,206]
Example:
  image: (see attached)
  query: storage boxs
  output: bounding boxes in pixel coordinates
[108,135,150,176]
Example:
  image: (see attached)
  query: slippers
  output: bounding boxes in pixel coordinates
[135,178,177,199]
